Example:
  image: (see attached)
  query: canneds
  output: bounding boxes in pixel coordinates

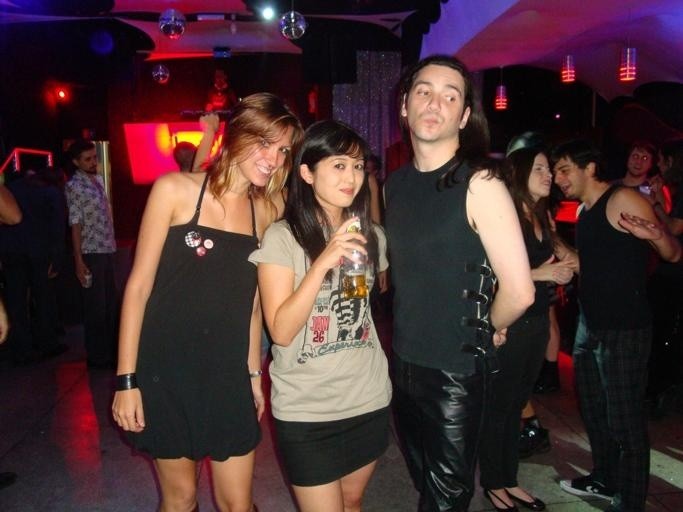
[84,271,93,289]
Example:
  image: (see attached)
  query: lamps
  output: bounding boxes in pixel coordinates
[619,6,637,81]
[495,63,509,110]
[159,8,186,35]
[280,0,306,39]
[560,43,576,83]
[152,62,171,81]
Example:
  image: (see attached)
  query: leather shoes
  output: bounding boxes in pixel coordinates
[483,489,518,512]
[504,488,546,512]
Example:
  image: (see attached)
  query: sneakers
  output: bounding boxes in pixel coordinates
[560,474,616,501]
[518,422,551,458]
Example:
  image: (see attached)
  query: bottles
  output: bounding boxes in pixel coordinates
[341,219,369,302]
[79,268,92,287]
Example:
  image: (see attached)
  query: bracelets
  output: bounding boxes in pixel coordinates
[115,373,139,391]
[250,370,262,378]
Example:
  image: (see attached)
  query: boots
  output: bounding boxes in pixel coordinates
[533,357,559,394]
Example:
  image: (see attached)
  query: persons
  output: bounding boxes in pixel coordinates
[479,141,683,511]
[383,55,536,512]
[112,93,305,511]
[2,142,120,486]
[364,156,386,224]
[173,114,217,172]
[247,122,393,509]
